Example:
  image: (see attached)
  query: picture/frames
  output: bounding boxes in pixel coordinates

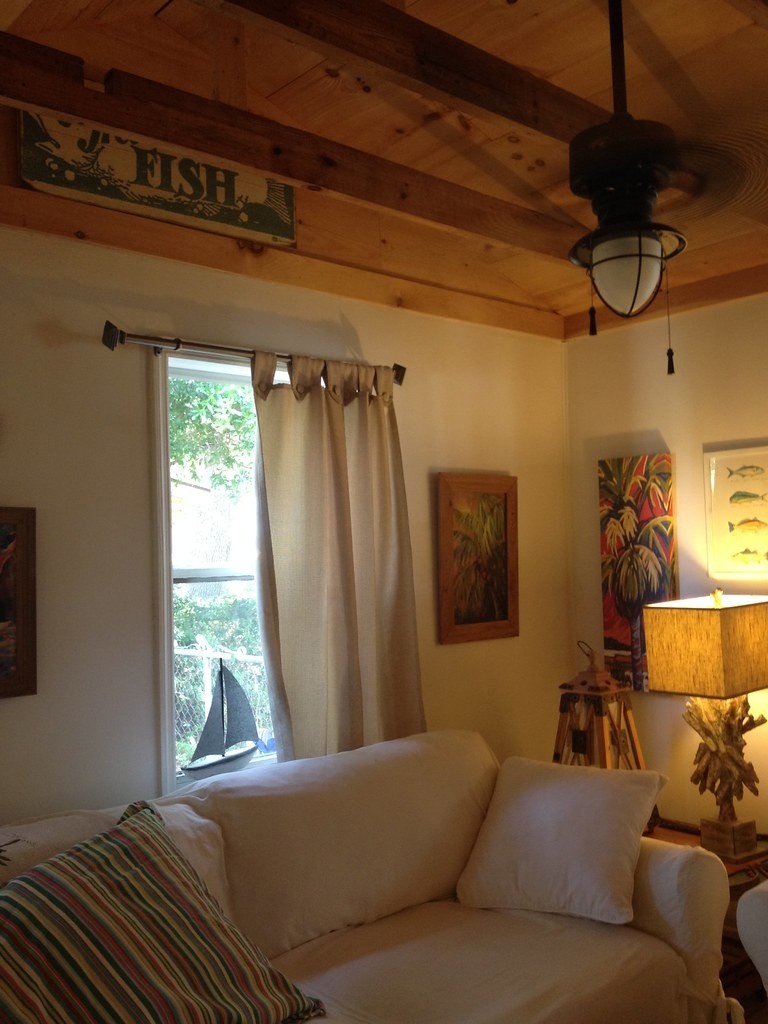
[0,506,37,700]
[435,471,521,645]
[703,445,768,582]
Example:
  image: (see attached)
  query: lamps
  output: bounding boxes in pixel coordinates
[642,588,768,866]
[569,0,686,375]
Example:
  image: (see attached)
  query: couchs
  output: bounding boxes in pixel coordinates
[0,727,744,1024]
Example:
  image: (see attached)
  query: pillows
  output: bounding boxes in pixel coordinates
[456,756,670,926]
[0,800,327,1024]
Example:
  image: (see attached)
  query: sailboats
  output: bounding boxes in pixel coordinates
[180,657,260,783]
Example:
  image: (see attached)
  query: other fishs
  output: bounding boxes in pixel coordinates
[732,549,768,564]
[728,517,768,533]
[729,491,768,504]
[726,465,765,479]
